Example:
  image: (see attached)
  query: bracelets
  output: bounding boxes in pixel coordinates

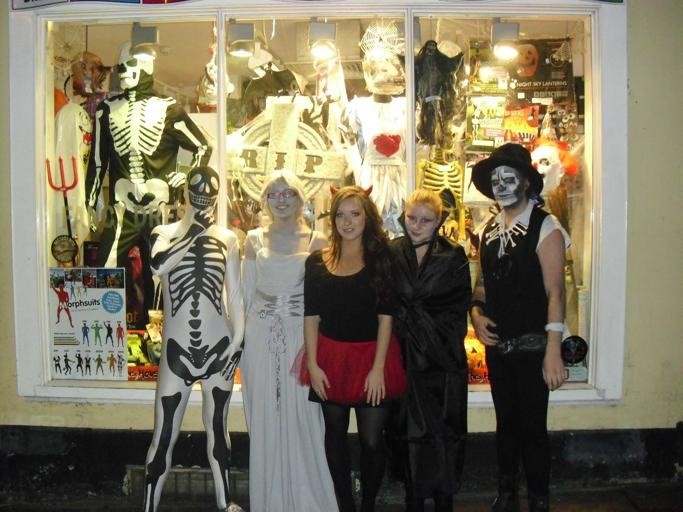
[469,298,486,316]
[544,320,566,334]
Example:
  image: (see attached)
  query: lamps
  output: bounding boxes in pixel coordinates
[226,20,254,59]
[490,19,520,61]
[395,19,422,58]
[306,18,337,59]
[126,24,161,61]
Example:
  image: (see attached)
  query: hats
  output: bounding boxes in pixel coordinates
[473,144,543,199]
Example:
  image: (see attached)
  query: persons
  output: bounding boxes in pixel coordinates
[141,165,248,511]
[391,188,472,508]
[414,123,466,202]
[349,47,412,183]
[85,42,213,326]
[235,167,338,510]
[47,270,125,376]
[466,142,566,509]
[55,53,104,238]
[303,180,392,511]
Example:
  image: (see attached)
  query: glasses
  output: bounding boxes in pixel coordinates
[268,189,296,199]
